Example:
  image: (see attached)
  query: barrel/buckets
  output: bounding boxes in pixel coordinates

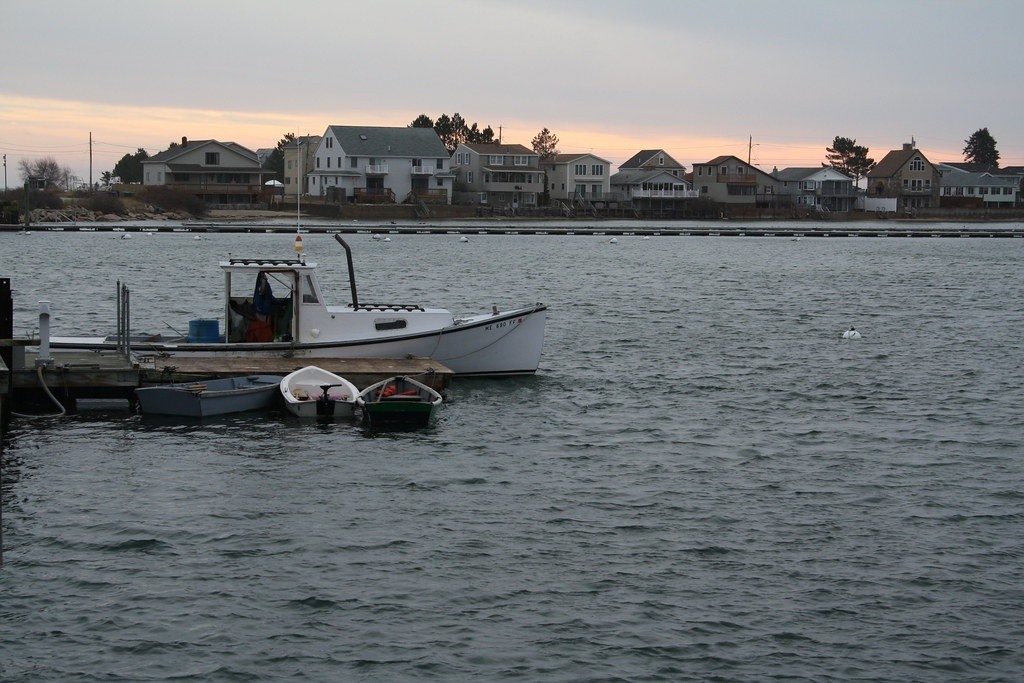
[189,320,219,343]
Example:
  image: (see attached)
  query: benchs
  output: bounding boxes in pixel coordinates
[377,396,423,401]
[240,385,259,389]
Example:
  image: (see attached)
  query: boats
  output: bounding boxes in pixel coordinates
[354,376,443,431]
[135,375,284,417]
[280,365,360,420]
[24,257,552,377]
